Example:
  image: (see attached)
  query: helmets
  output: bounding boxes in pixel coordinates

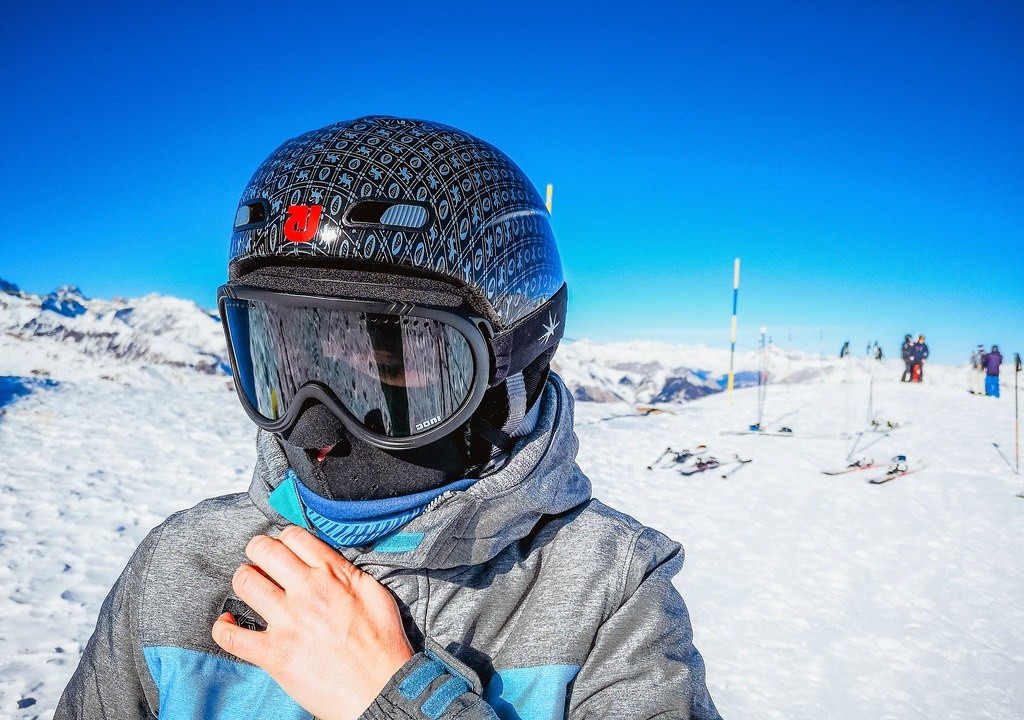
[229,115,569,450]
[992,345,999,352]
[905,334,912,341]
[919,336,925,341]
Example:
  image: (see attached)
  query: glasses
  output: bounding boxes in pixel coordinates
[216,266,498,452]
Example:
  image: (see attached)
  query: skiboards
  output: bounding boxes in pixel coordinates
[720,416,917,438]
[658,445,739,476]
[821,452,923,484]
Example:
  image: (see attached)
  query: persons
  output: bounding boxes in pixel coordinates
[900,334,914,383]
[839,339,986,370]
[981,344,1002,397]
[51,113,722,720]
[913,336,928,382]
